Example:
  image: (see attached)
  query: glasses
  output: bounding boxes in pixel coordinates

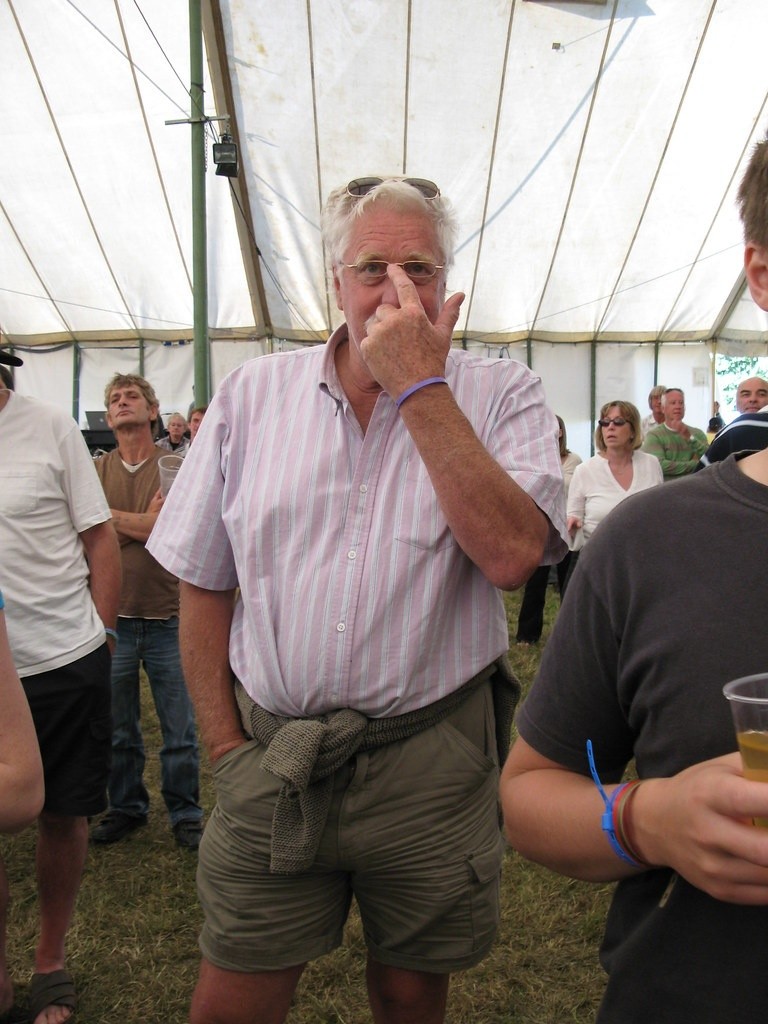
[598,419,631,427]
[344,177,441,203]
[340,260,445,278]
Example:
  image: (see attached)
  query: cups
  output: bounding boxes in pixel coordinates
[722,673,768,829]
[157,455,185,498]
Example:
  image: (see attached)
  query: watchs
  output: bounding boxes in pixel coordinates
[688,434,695,440]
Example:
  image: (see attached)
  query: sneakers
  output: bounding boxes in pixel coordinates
[93,810,148,844]
[175,817,203,847]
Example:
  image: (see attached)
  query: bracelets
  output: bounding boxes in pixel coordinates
[105,627,119,640]
[395,377,449,410]
[587,737,645,868]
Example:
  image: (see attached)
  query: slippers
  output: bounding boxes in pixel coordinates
[0,1007,31,1024]
[28,969,77,1024]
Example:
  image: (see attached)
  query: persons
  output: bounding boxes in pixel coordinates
[694,376,768,474]
[140,174,572,1024]
[498,134,768,1024]
[565,400,664,544]
[92,370,208,851]
[0,351,125,1024]
[643,388,708,484]
[514,412,583,647]
[150,407,208,464]
[638,386,669,450]
